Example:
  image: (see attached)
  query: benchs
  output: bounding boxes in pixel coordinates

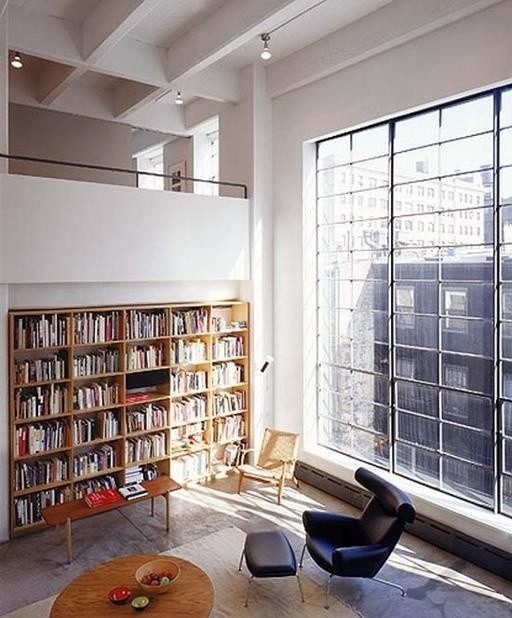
[40,475,182,563]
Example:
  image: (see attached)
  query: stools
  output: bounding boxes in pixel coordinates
[237,531,305,608]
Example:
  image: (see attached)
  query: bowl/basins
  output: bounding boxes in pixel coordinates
[108,587,131,605]
[135,560,181,594]
[130,597,149,610]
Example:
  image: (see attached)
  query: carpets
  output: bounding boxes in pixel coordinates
[0,525,365,618]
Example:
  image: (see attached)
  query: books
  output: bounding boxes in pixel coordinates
[14,308,247,523]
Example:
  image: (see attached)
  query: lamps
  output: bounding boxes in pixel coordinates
[175,91,184,104]
[10,52,24,69]
[260,34,273,61]
[260,356,273,375]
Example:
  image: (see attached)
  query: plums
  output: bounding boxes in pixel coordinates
[142,571,173,586]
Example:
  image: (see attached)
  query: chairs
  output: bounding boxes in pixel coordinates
[230,428,302,505]
[299,466,416,610]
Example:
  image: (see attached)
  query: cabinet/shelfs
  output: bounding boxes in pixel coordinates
[8,299,250,540]
